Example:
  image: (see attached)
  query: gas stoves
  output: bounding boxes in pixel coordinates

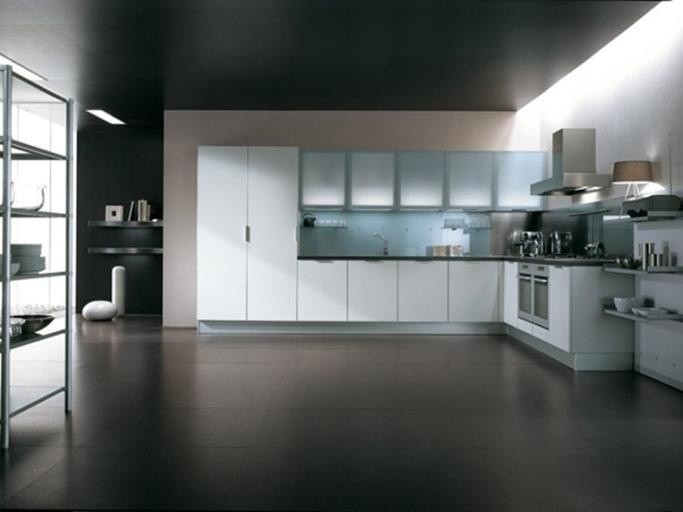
[536,253,590,258]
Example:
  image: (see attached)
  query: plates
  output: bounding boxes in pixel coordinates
[631,308,667,318]
[10,244,45,275]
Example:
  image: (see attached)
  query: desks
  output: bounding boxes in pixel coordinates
[86,220,163,317]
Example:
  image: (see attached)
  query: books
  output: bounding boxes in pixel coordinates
[126,199,151,223]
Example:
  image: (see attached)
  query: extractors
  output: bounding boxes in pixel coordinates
[529,127,611,198]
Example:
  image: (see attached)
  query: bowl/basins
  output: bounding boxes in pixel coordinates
[10,314,54,334]
[613,297,633,313]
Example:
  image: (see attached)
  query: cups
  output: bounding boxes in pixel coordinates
[317,220,347,227]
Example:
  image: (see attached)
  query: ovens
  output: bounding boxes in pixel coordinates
[517,263,549,330]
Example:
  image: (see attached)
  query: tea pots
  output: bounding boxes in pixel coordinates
[303,213,316,227]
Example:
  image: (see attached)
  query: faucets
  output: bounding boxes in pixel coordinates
[374,233,389,255]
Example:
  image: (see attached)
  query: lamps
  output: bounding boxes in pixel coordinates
[613,158,655,219]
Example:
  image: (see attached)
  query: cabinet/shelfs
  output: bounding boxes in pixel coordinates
[548,267,634,371]
[348,146,396,213]
[398,259,449,335]
[349,258,398,334]
[502,261,519,342]
[398,150,440,213]
[196,145,298,335]
[494,150,548,213]
[0,64,75,450]
[600,263,682,324]
[300,145,346,210]
[298,256,348,333]
[449,262,498,335]
[444,149,492,210]
